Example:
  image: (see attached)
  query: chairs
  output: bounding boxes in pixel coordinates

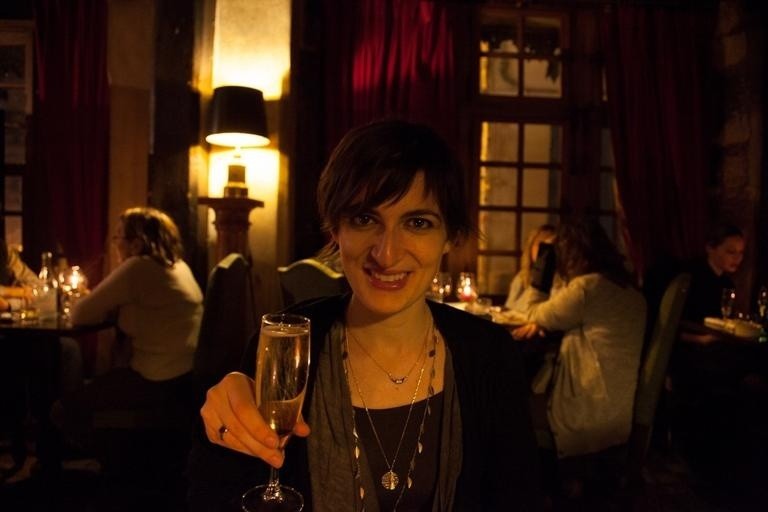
[76,252,277,486]
[540,276,685,510]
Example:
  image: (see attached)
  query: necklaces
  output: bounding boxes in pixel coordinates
[341,319,439,512]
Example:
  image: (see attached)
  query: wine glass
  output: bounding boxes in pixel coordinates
[240,313,311,512]
[721,288,735,326]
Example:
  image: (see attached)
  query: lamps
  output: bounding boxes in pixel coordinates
[203,87,271,197]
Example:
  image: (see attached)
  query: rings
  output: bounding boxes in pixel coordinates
[219,425,229,440]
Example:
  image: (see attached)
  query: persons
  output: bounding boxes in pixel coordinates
[70,208,205,429]
[658,221,743,462]
[1,209,39,313]
[186,116,558,512]
[506,223,647,461]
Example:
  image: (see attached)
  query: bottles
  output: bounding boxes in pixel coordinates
[36,250,57,316]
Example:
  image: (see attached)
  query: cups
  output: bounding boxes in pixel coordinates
[431,272,476,304]
